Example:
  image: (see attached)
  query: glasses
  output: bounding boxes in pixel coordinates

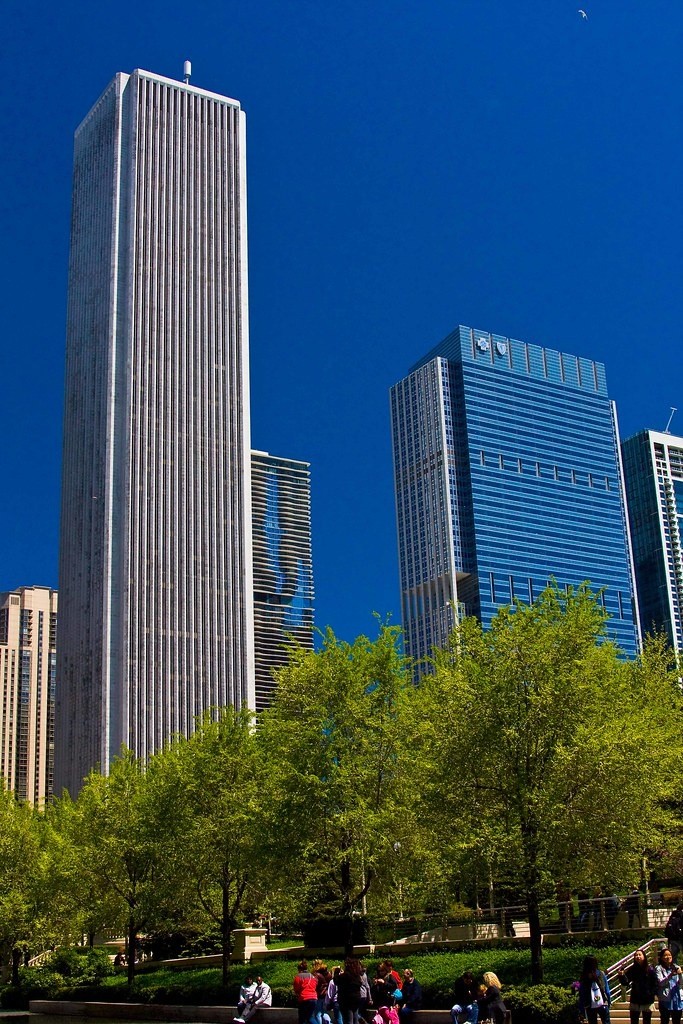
[377,969,385,973]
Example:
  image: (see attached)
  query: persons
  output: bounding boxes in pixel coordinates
[232,976,272,1024]
[23,946,32,967]
[617,950,658,1024]
[603,886,621,930]
[655,949,683,1024]
[664,903,683,964]
[623,887,642,928]
[450,972,507,1024]
[293,956,422,1024]
[114,952,125,966]
[577,889,589,930]
[577,955,611,1024]
[592,887,603,930]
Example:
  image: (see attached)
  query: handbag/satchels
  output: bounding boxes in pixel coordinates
[326,1000,334,1012]
[393,989,402,1000]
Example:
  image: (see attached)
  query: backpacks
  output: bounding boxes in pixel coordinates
[586,973,607,1009]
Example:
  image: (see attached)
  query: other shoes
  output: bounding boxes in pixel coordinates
[463,1021,472,1024]
[233,1017,245,1024]
